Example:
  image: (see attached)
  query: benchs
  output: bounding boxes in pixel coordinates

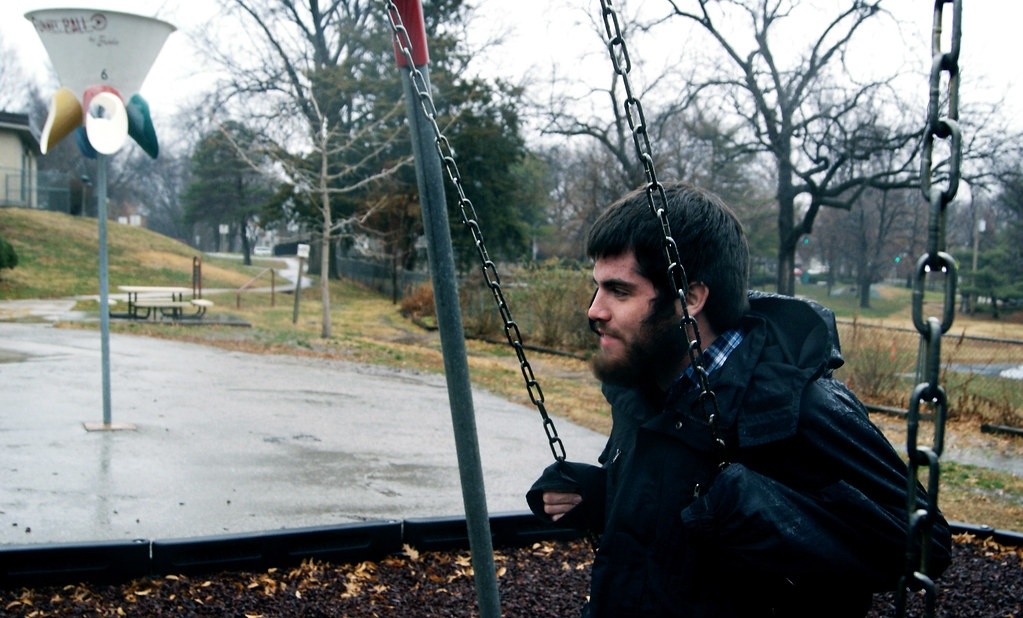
[96,298,215,319]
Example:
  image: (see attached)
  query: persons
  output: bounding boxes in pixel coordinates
[527,183,952,618]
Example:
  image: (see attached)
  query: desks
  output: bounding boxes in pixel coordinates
[118,285,194,322]
